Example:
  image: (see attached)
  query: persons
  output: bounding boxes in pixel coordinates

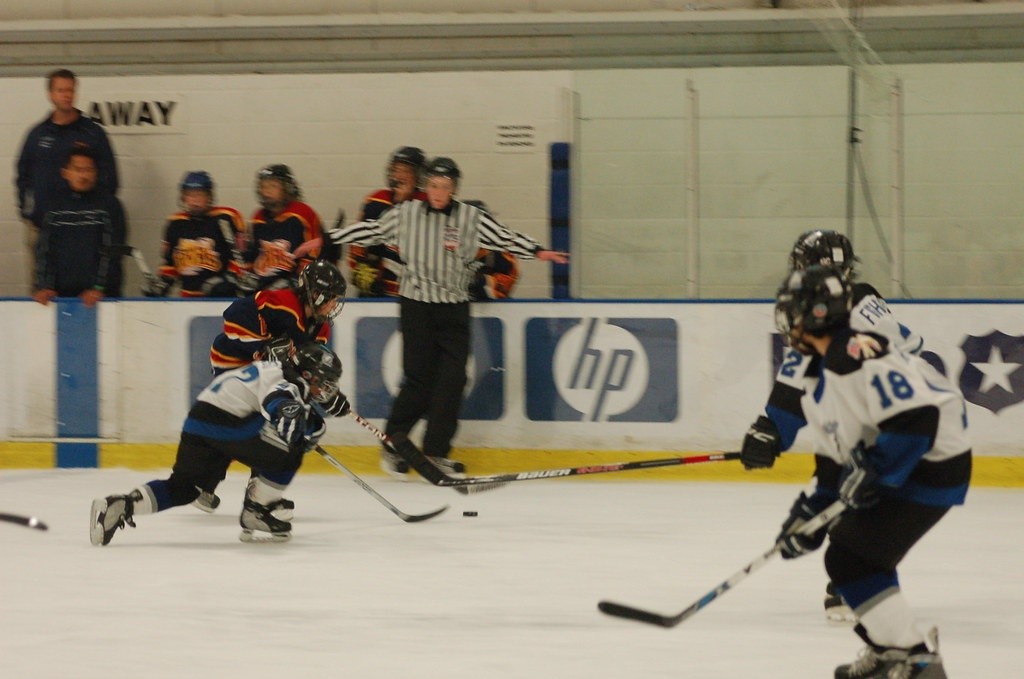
[142,163,325,297]
[774,264,972,679]
[349,145,429,301]
[89,341,342,547]
[29,146,126,306]
[459,201,518,300]
[290,158,571,486]
[190,262,350,523]
[15,69,120,231]
[741,231,949,620]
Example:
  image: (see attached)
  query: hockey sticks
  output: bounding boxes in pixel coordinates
[597,499,850,628]
[124,245,160,297]
[0,513,48,531]
[303,409,741,524]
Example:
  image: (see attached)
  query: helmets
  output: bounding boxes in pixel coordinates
[393,147,423,167]
[297,258,346,321]
[791,230,852,279]
[777,266,848,354]
[427,157,461,180]
[290,344,342,403]
[183,172,211,189]
[258,164,292,183]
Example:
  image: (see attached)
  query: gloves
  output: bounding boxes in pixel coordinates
[839,438,884,510]
[775,494,830,560]
[740,417,780,470]
[319,391,350,417]
[270,398,327,456]
[253,337,296,365]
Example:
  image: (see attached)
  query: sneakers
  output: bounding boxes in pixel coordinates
[263,498,294,522]
[89,494,136,547]
[834,623,947,679]
[191,486,220,513]
[238,482,291,543]
[380,453,408,480]
[425,455,463,474]
[825,598,858,623]
